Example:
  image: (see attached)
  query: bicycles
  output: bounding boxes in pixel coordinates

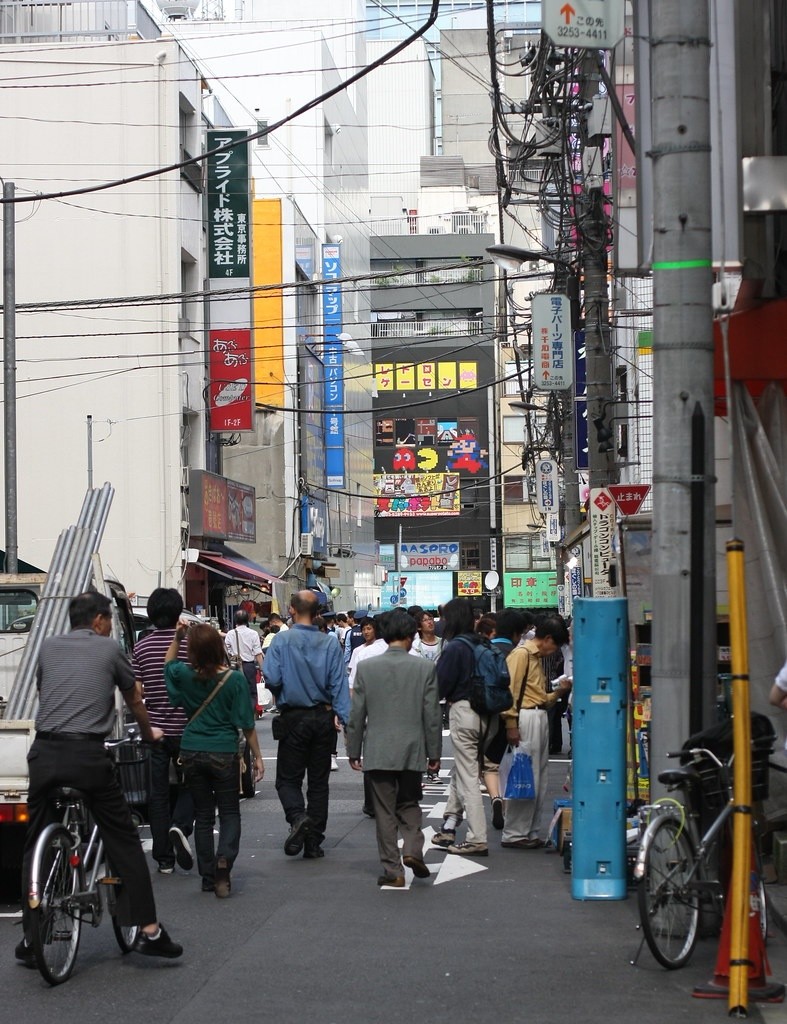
[25,727,166,987]
[629,735,776,970]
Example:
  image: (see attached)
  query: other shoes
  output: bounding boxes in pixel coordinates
[361,805,376,818]
[477,778,487,791]
[427,773,444,783]
[501,837,552,848]
[169,826,194,871]
[303,843,326,858]
[157,861,175,874]
[330,754,339,770]
[284,813,313,856]
[201,875,215,892]
[212,854,232,898]
[490,796,504,830]
[402,856,430,878]
[378,873,406,887]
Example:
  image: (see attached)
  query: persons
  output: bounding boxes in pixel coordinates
[499,614,572,850]
[163,621,265,897]
[131,590,195,874]
[431,599,500,857]
[268,611,449,816]
[263,589,353,859]
[15,595,183,967]
[346,608,443,887]
[520,621,573,759]
[479,612,514,831]
[768,656,787,712]
[225,608,265,715]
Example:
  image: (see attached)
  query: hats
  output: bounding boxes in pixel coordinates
[321,612,336,618]
[354,610,368,618]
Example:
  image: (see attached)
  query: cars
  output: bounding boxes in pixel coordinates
[131,603,260,799]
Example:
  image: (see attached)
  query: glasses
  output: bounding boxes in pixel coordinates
[422,616,435,622]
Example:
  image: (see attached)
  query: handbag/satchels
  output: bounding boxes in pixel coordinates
[227,657,240,670]
[256,676,273,708]
[498,738,538,801]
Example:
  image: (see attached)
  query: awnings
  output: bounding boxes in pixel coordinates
[201,546,292,615]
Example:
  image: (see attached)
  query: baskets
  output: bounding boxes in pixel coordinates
[682,736,776,810]
[106,737,151,812]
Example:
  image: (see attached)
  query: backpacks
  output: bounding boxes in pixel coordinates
[454,632,515,715]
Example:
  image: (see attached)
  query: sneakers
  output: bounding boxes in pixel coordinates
[447,841,490,856]
[431,823,457,847]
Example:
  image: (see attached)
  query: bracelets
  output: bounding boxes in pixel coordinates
[251,753,262,764]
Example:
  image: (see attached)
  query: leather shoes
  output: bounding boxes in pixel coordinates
[13,936,44,961]
[134,923,184,958]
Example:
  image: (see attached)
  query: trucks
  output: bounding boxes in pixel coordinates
[0,570,142,897]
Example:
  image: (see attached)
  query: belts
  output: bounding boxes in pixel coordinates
[525,704,547,710]
